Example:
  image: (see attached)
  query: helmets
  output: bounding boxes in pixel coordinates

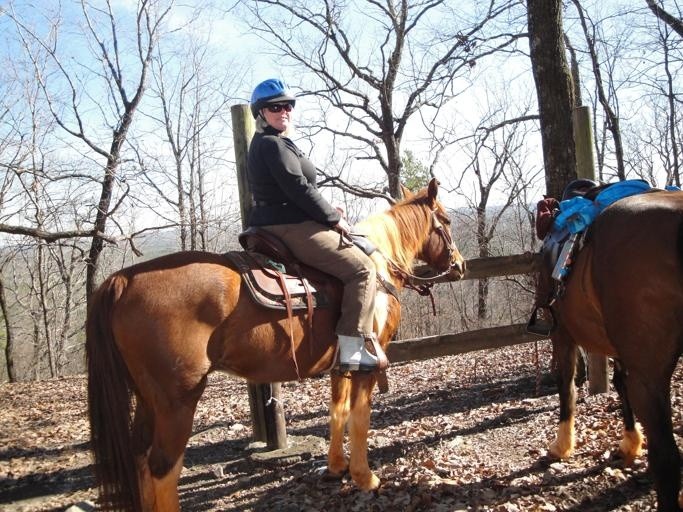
[251,79,296,119]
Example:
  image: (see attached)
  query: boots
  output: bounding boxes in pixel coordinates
[338,335,378,372]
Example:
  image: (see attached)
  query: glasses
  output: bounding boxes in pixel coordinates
[264,103,292,113]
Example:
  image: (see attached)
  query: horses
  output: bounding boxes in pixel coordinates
[84,177,465,511]
[538,188,683,511]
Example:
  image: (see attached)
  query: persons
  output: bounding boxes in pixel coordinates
[246,77,390,372]
